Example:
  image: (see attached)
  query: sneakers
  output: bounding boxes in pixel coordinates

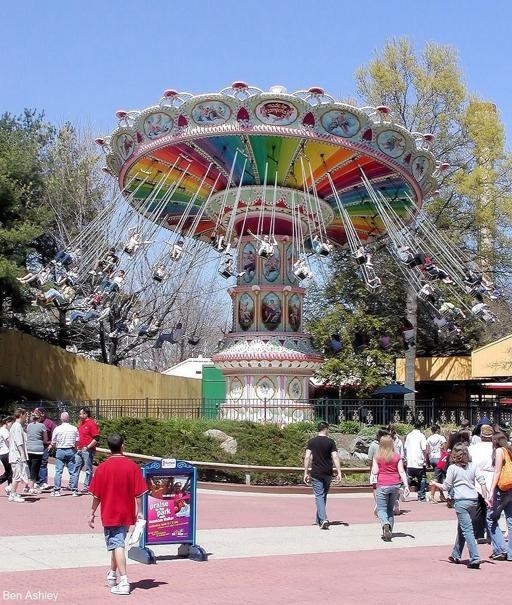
[110,583,130,595]
[5,482,89,502]
[320,520,329,529]
[106,570,116,587]
[373,488,507,568]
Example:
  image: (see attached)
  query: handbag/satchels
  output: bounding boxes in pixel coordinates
[497,463,512,492]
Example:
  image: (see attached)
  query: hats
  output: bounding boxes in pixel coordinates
[481,425,494,438]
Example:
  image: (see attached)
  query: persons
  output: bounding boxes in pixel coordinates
[108,313,200,348]
[247,229,278,257]
[303,422,342,529]
[211,232,246,277]
[462,266,502,323]
[312,236,333,254]
[87,247,120,275]
[355,246,385,298]
[327,334,342,355]
[416,282,467,343]
[0,406,101,503]
[380,317,413,353]
[163,240,193,261]
[18,248,81,287]
[367,418,512,569]
[65,270,125,326]
[153,262,170,281]
[164,482,191,514]
[87,433,150,594]
[396,244,456,286]
[294,259,313,282]
[32,284,77,307]
[127,228,156,253]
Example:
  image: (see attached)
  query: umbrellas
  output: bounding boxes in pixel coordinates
[369,382,414,398]
